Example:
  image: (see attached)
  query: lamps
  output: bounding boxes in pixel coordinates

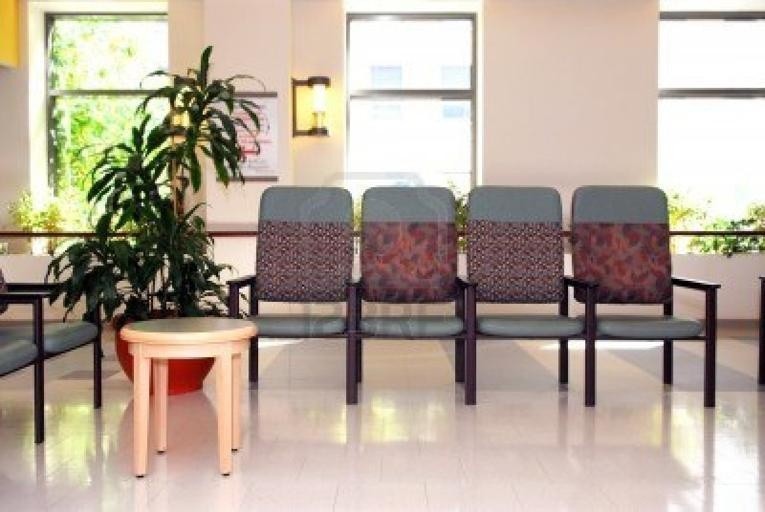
[292,76,330,138]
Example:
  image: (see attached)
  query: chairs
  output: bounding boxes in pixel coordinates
[0,267,102,443]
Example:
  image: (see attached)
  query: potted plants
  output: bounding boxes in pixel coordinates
[44,47,270,395]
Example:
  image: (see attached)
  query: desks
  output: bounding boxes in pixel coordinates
[119,316,258,479]
